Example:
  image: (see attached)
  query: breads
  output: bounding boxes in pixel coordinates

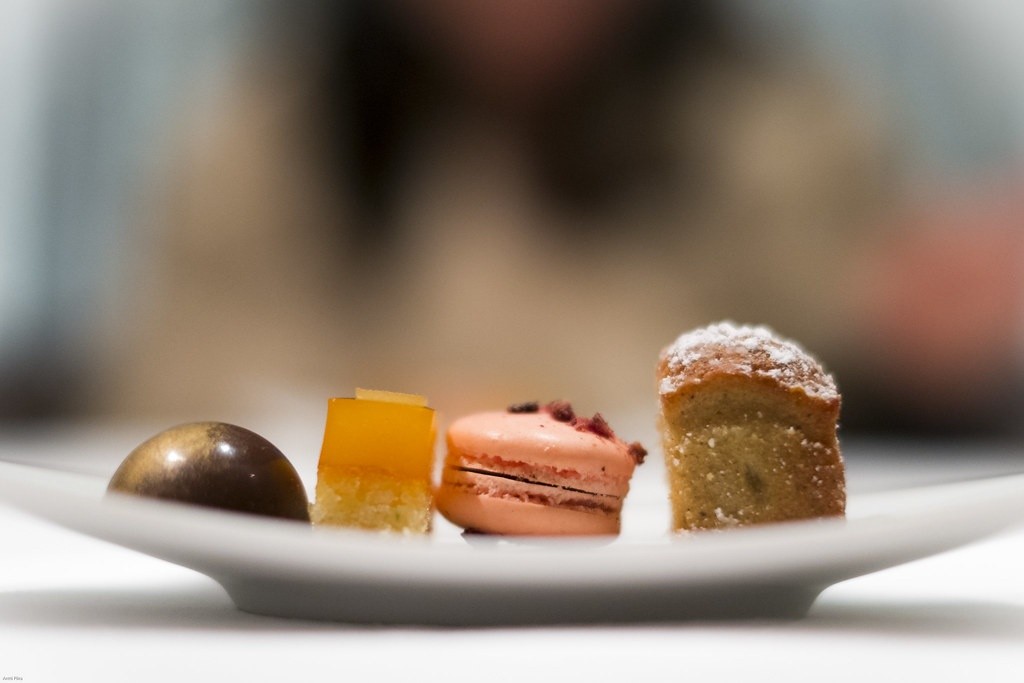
[658,320,846,531]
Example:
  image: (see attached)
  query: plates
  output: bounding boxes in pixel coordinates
[1,460,1022,619]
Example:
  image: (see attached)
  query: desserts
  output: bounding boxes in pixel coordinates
[432,401,648,537]
[103,419,310,521]
[309,385,440,534]
